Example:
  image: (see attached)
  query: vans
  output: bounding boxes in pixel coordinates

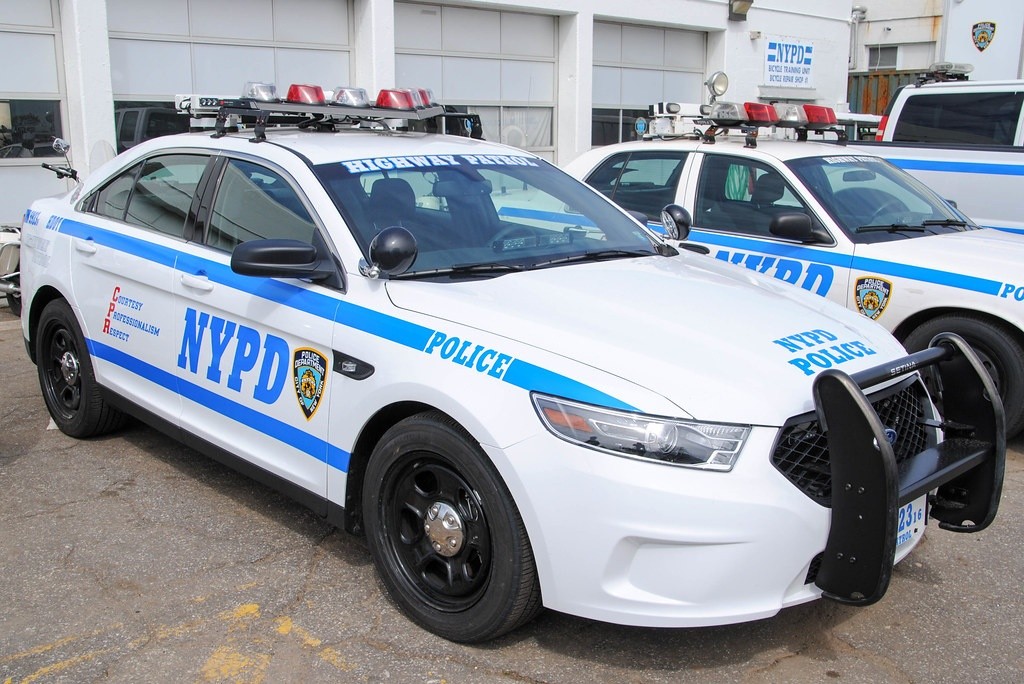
[115,106,190,155]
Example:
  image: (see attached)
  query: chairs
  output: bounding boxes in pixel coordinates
[367,178,425,255]
[749,172,792,236]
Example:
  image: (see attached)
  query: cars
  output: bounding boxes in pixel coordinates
[416,76,1024,449]
[17,83,1006,645]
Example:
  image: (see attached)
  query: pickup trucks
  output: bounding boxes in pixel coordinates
[754,60,1024,235]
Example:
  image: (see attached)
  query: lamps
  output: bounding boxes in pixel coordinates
[728,0,753,21]
